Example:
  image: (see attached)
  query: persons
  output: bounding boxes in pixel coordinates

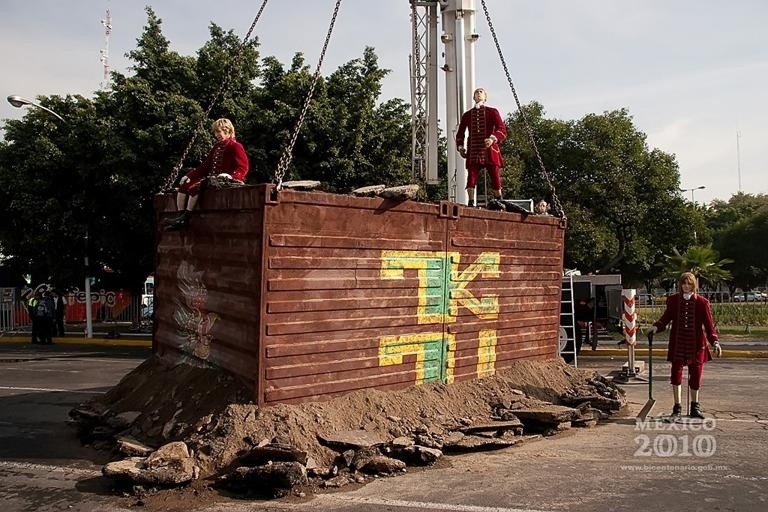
[645,273,721,422]
[24,289,66,344]
[454,87,507,210]
[162,117,251,230]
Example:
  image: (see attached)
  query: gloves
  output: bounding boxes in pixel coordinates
[643,325,659,338]
[712,341,723,360]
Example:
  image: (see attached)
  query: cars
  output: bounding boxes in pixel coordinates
[635,290,768,304]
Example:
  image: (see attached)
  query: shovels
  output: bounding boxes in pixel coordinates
[634,329,656,425]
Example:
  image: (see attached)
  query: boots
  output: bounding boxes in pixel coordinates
[690,401,706,420]
[664,403,684,425]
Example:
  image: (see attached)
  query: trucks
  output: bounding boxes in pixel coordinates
[557,271,624,364]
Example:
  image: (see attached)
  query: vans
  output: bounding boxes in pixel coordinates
[139,274,156,316]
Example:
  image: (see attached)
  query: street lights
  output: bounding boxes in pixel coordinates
[678,185,706,243]
[4,94,95,340]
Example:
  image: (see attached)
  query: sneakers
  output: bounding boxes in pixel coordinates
[162,208,191,234]
